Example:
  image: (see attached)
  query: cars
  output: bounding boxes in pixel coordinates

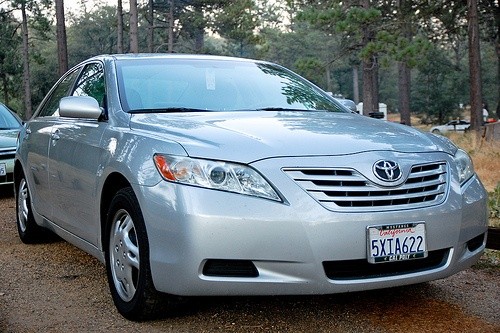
[0,101,27,189]
[13,52,491,320]
[429,119,471,135]
